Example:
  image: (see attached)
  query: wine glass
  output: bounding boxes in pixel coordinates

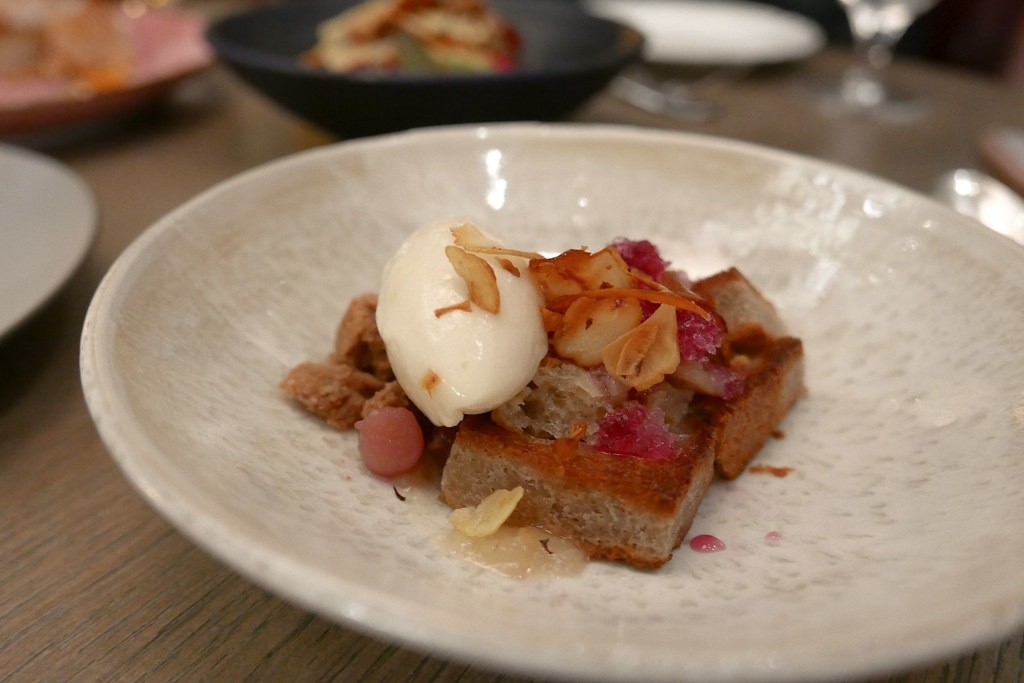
[814,1,937,116]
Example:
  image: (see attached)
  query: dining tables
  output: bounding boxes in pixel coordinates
[1,38,1023,681]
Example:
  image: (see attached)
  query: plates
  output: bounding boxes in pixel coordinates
[588,0,824,73]
[0,139,101,337]
[77,119,1024,683]
[207,1,636,142]
[0,19,224,145]
